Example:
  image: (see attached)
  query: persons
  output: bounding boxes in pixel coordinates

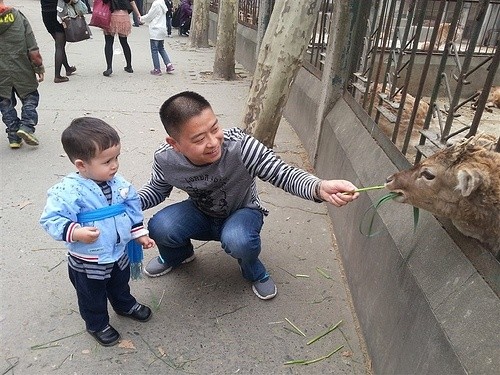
[132,0,144,27]
[100,0,142,76]
[38,118,155,348]
[165,0,193,37]
[82,0,93,14]
[138,0,176,75]
[136,91,360,300]
[38,0,77,83]
[0,0,45,149]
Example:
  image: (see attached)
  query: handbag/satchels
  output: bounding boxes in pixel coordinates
[61,4,90,42]
[88,0,112,30]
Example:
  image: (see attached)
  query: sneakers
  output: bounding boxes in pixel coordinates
[115,301,152,322]
[9,136,24,148]
[144,250,195,278]
[166,63,175,74]
[16,124,39,145]
[151,69,162,76]
[86,323,120,347]
[240,266,278,299]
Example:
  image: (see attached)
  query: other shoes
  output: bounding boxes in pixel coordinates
[124,67,133,73]
[103,70,112,76]
[66,66,76,76]
[54,76,69,83]
[168,34,173,38]
[132,21,145,27]
[181,32,189,37]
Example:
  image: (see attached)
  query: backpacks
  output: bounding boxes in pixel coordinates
[172,2,188,27]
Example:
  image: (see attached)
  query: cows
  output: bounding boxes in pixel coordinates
[384,133,500,257]
[471,86,500,113]
